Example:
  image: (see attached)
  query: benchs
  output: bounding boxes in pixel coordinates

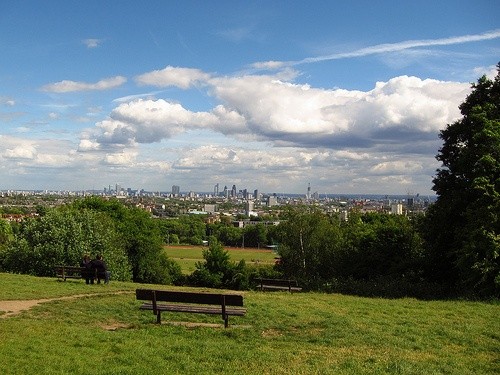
[136,289,247,327]
[251,275,303,294]
[54,265,112,284]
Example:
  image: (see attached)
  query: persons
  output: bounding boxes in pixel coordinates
[80,255,96,284]
[92,254,111,285]
